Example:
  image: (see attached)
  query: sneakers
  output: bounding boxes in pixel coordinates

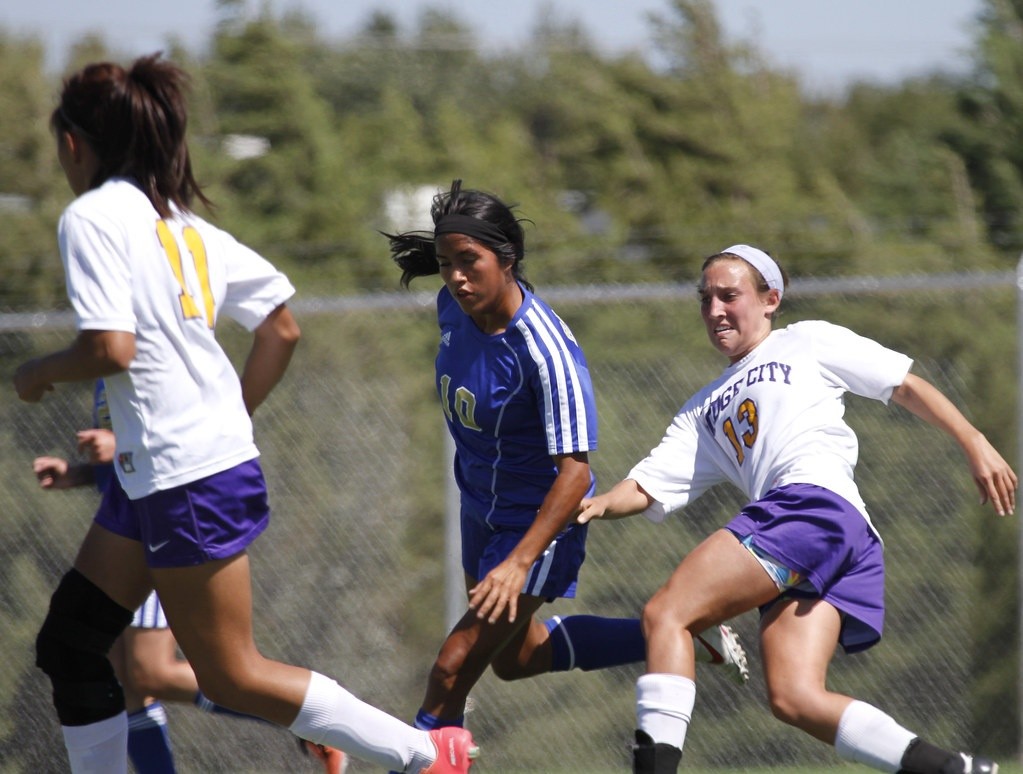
[300,738,347,774]
[959,751,999,774]
[419,726,481,774]
[694,623,750,687]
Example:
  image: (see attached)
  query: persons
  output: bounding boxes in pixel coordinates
[10,51,482,774]
[536,245,1020,774]
[376,180,749,774]
